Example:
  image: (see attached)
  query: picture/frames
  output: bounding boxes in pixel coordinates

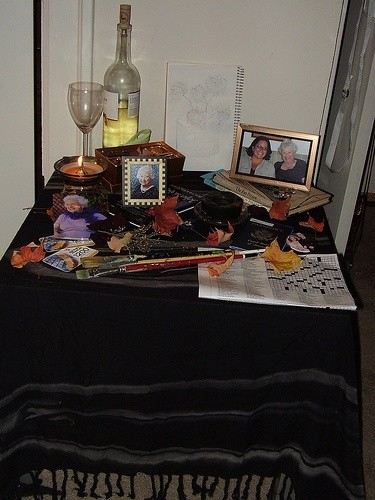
[229,123,319,192]
[122,156,167,207]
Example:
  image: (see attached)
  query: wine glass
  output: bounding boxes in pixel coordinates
[67,81,105,158]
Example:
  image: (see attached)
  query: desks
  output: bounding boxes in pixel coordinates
[0,156,366,500]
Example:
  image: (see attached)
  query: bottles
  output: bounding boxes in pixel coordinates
[102,4,141,148]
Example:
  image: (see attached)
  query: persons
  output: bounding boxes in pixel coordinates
[131,165,159,199]
[274,141,307,185]
[238,136,275,178]
[54,195,107,237]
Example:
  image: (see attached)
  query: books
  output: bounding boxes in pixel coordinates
[200,169,331,216]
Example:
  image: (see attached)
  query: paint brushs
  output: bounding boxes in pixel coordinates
[76,229,262,282]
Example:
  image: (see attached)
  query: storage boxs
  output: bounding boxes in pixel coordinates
[95,141,186,192]
